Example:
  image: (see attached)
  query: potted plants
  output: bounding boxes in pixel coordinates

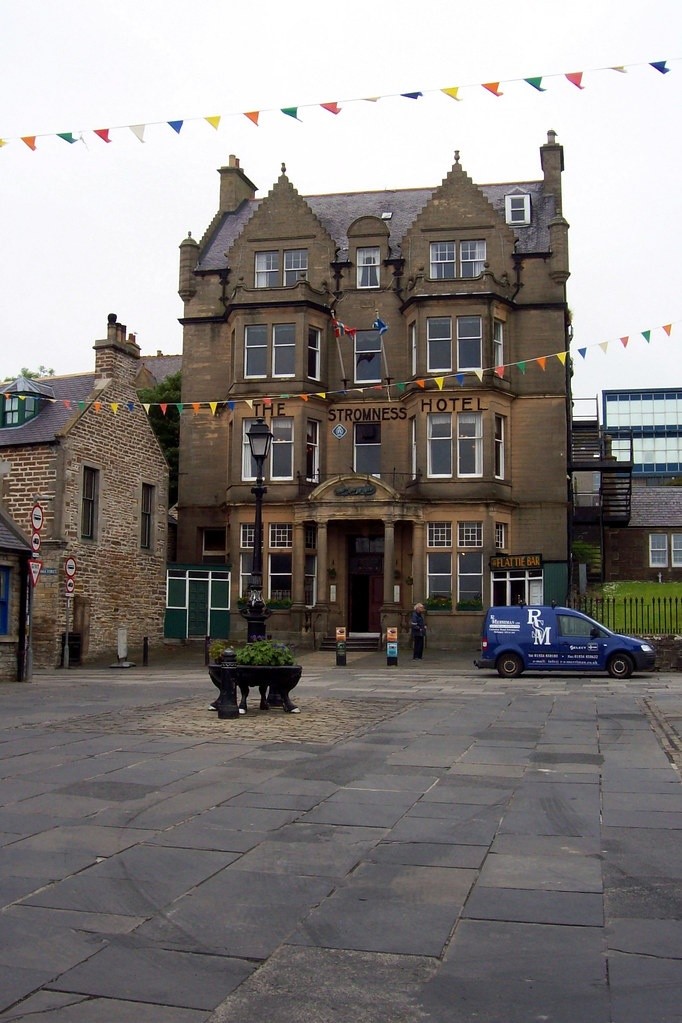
[456,598,482,611]
[423,593,452,611]
[238,597,292,609]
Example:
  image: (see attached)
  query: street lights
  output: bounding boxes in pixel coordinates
[239,417,275,642]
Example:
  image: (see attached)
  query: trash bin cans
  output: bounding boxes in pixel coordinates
[62,633,82,667]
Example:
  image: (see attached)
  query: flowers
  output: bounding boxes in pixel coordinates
[205,634,300,666]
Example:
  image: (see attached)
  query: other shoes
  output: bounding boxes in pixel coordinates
[413,657,423,662]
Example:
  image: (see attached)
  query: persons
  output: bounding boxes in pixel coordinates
[411,603,427,661]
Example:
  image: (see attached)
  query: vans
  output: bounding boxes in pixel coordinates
[473,599,657,679]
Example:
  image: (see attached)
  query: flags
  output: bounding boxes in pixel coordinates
[331,319,356,339]
[372,318,388,335]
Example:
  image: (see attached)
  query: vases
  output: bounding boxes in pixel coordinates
[207,663,303,713]
